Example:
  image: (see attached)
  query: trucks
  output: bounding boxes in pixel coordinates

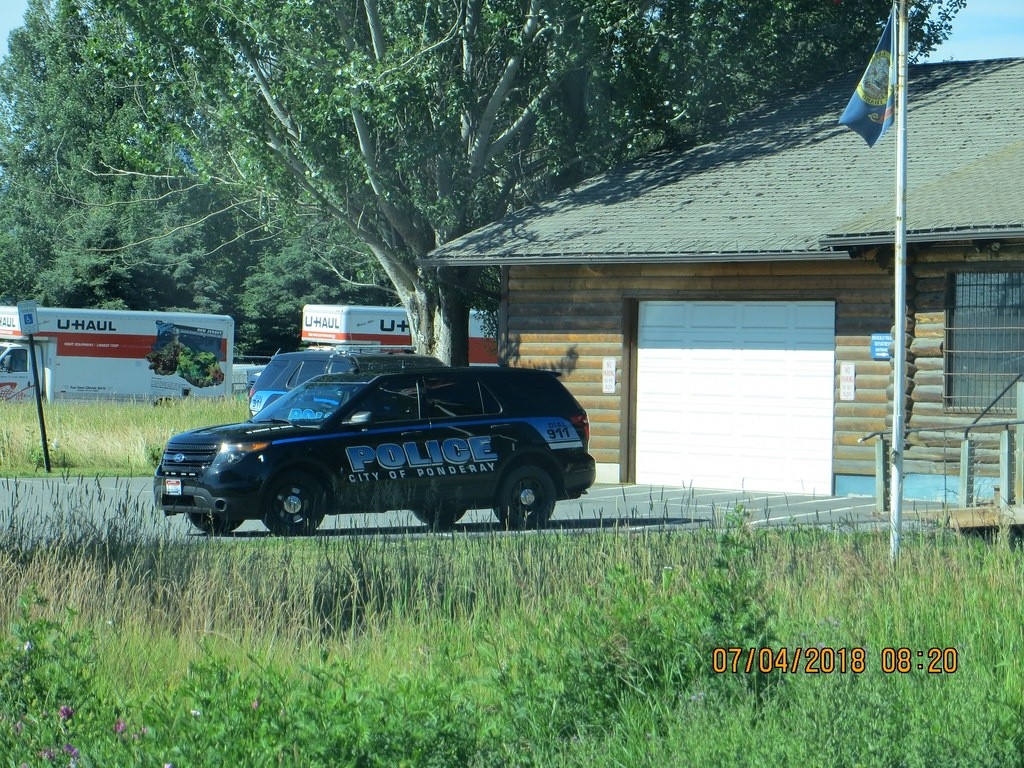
[0,304,235,408]
[300,303,499,365]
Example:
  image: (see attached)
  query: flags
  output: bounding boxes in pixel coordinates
[838,0,899,150]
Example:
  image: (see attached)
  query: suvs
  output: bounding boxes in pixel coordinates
[248,342,456,425]
[152,360,597,537]
